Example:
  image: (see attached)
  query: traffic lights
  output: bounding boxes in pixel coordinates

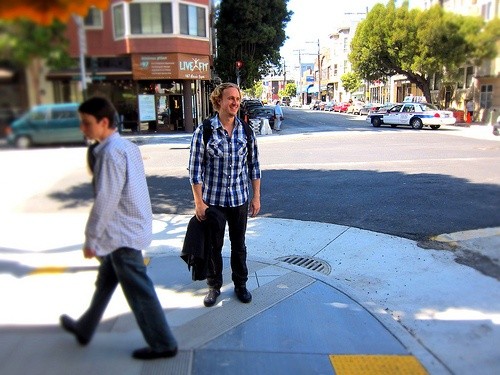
[236,62,241,67]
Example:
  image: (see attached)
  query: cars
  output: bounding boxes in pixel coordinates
[309,100,386,116]
[240,99,275,129]
[366,103,457,130]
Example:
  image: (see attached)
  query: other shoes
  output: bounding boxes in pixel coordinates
[61,314,90,346]
[234,286,252,303]
[204,289,221,307]
[132,345,178,360]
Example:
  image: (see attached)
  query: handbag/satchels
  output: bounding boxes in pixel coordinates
[280,117,284,121]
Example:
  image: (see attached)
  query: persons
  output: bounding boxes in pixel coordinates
[274,101,283,131]
[189,82,262,307]
[63,98,177,360]
[467,98,474,121]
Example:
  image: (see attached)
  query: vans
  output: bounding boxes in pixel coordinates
[5,102,97,149]
